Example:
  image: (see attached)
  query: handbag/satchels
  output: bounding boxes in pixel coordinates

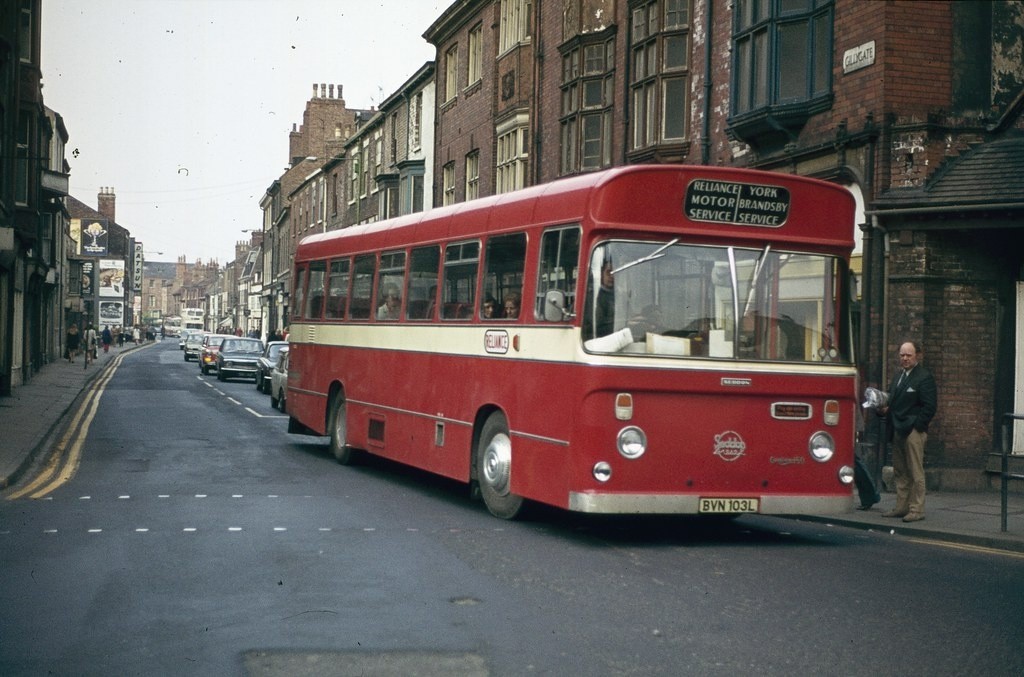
[64,348,69,359]
[92,339,96,344]
[93,346,98,359]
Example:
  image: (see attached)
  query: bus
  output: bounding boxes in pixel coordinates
[180,308,205,332]
[286,164,884,533]
[164,317,182,337]
[144,317,162,333]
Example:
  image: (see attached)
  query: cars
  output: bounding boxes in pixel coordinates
[184,333,203,361]
[198,333,235,376]
[270,346,289,413]
[215,337,264,382]
[255,341,289,395]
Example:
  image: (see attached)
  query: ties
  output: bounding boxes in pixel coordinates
[898,371,906,386]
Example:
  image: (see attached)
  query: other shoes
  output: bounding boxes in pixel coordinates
[69,357,71,361]
[87,358,90,363]
[901,511,925,522]
[882,505,909,517]
[858,494,880,511]
[72,360,74,363]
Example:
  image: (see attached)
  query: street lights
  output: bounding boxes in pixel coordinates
[241,229,275,339]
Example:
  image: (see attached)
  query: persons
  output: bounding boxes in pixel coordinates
[215,325,289,342]
[583,256,646,342]
[504,292,521,320]
[140,324,166,343]
[66,323,79,364]
[854,451,881,511]
[132,326,141,346]
[483,297,495,319]
[83,324,97,365]
[111,324,124,348]
[102,325,113,353]
[873,339,938,522]
[375,282,401,321]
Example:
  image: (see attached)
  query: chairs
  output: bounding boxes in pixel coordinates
[310,295,470,320]
